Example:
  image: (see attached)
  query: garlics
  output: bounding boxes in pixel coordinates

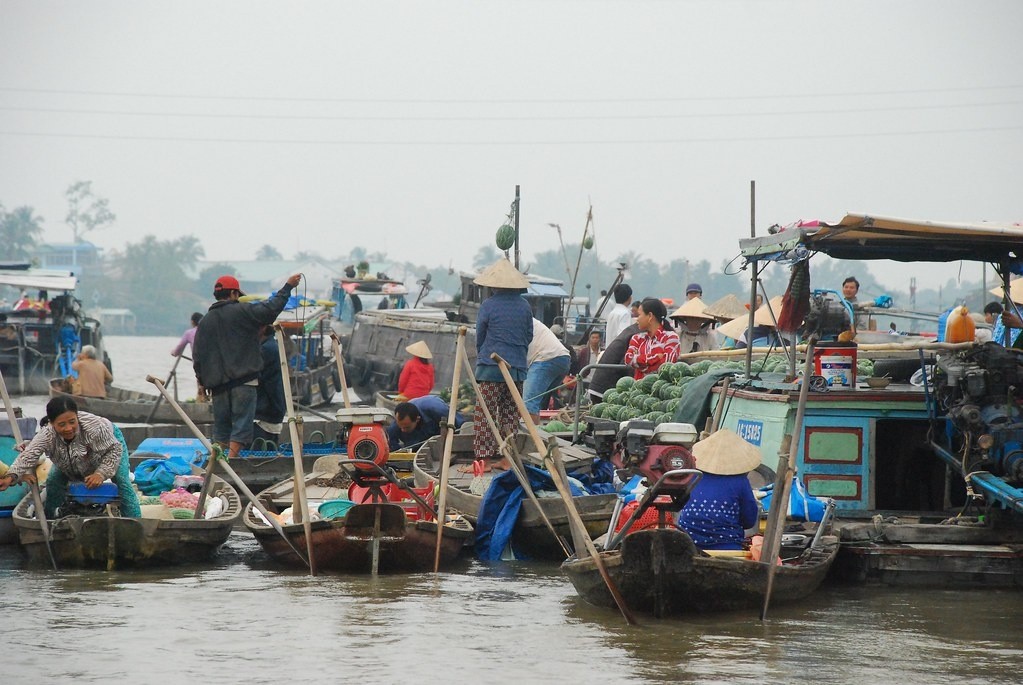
[160,492,198,509]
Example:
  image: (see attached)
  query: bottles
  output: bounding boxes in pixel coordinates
[938,306,976,342]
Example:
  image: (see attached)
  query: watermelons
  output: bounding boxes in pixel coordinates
[140,497,162,504]
[536,420,588,432]
[589,354,873,423]
[583,237,593,249]
[169,507,195,519]
[496,224,516,250]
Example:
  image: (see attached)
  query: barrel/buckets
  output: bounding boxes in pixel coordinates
[347,477,389,504]
[814,347,857,389]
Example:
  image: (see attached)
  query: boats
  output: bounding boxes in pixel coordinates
[559,528,839,618]
[48,377,214,424]
[12,452,241,571]
[240,296,337,408]
[413,434,618,553]
[0,262,112,397]
[243,455,474,570]
[329,186,628,405]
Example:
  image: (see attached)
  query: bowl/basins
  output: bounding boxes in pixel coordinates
[781,535,806,546]
[865,378,892,390]
[317,501,357,520]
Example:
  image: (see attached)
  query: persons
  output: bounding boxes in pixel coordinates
[605,284,637,352]
[456,257,537,474]
[0,395,144,518]
[576,328,603,391]
[677,426,760,552]
[71,345,113,400]
[252,321,287,450]
[670,294,800,359]
[549,313,580,377]
[395,340,435,403]
[630,300,642,318]
[984,298,1003,331]
[193,275,303,456]
[386,394,467,456]
[685,283,703,300]
[839,276,861,332]
[170,312,212,400]
[521,315,572,430]
[596,290,608,313]
[622,296,682,381]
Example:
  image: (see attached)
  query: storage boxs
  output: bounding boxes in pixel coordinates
[386,481,434,522]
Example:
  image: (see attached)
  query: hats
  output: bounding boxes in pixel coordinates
[691,427,763,476]
[686,283,702,294]
[754,295,805,326]
[989,277,1023,304]
[472,256,531,288]
[669,297,716,320]
[717,313,751,340]
[214,276,247,297]
[702,294,749,319]
[406,340,432,359]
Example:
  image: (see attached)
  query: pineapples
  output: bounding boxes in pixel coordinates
[439,379,478,413]
